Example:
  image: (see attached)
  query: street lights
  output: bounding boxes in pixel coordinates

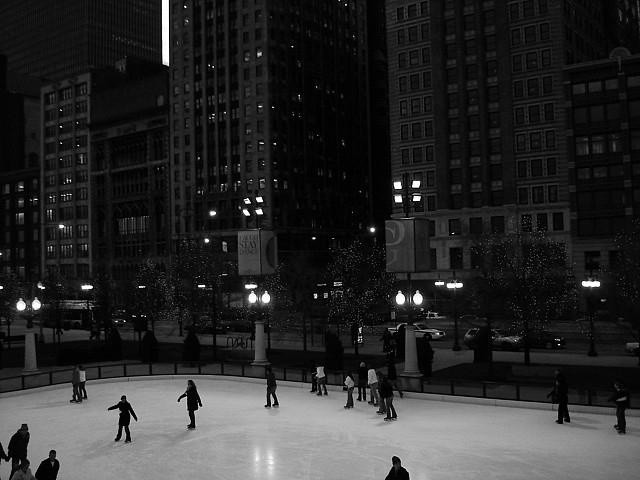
[16,295,44,375]
[246,290,274,377]
[435,280,445,309]
[197,283,207,315]
[202,238,219,344]
[58,222,81,280]
[447,279,463,351]
[80,284,94,328]
[209,209,219,306]
[138,282,148,315]
[580,278,601,358]
[36,281,46,301]
[393,171,425,290]
[242,195,263,293]
[395,288,426,394]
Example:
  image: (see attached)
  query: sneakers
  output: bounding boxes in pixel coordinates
[265,403,279,407]
[309,389,399,422]
[69,395,88,404]
[115,437,132,443]
[187,424,196,429]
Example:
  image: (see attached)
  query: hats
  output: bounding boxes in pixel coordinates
[392,456,401,465]
[21,424,28,431]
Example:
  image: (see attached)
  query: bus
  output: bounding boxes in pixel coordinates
[39,299,94,329]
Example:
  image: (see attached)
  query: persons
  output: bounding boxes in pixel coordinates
[310,361,328,396]
[264,366,280,408]
[79,365,88,399]
[70,363,82,403]
[356,361,398,421]
[9,459,36,480]
[35,448,60,479]
[177,379,203,429]
[0,441,10,469]
[107,394,137,443]
[343,370,355,408]
[384,456,409,480]
[546,373,570,424]
[7,422,31,471]
[606,381,630,434]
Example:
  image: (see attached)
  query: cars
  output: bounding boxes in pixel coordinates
[625,341,640,355]
[387,323,445,340]
[464,328,566,349]
[112,305,145,329]
[387,309,448,319]
[186,308,272,335]
